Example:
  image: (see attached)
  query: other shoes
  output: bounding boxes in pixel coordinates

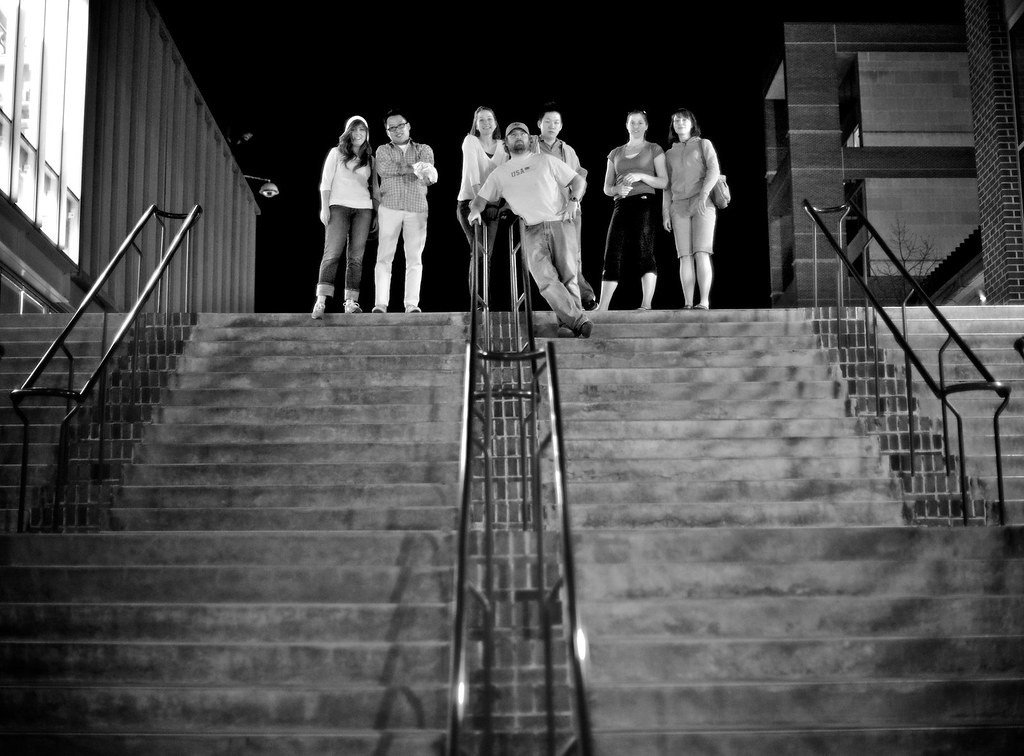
[477,305,484,311]
[683,304,710,309]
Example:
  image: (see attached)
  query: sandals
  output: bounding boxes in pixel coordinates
[637,306,651,310]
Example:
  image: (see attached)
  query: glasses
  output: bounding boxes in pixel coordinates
[385,121,409,132]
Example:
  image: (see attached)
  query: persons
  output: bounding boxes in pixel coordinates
[371,109,438,313]
[457,106,510,311]
[594,108,669,310]
[530,109,598,311]
[311,115,380,319]
[663,108,731,311]
[467,122,594,337]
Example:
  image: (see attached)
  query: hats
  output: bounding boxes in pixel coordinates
[505,122,529,136]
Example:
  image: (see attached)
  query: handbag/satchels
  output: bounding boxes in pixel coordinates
[697,139,731,209]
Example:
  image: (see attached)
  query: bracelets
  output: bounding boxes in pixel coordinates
[568,197,578,203]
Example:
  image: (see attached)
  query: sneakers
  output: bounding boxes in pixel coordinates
[311,302,325,320]
[405,305,421,313]
[372,305,386,314]
[343,301,362,313]
[557,320,593,338]
